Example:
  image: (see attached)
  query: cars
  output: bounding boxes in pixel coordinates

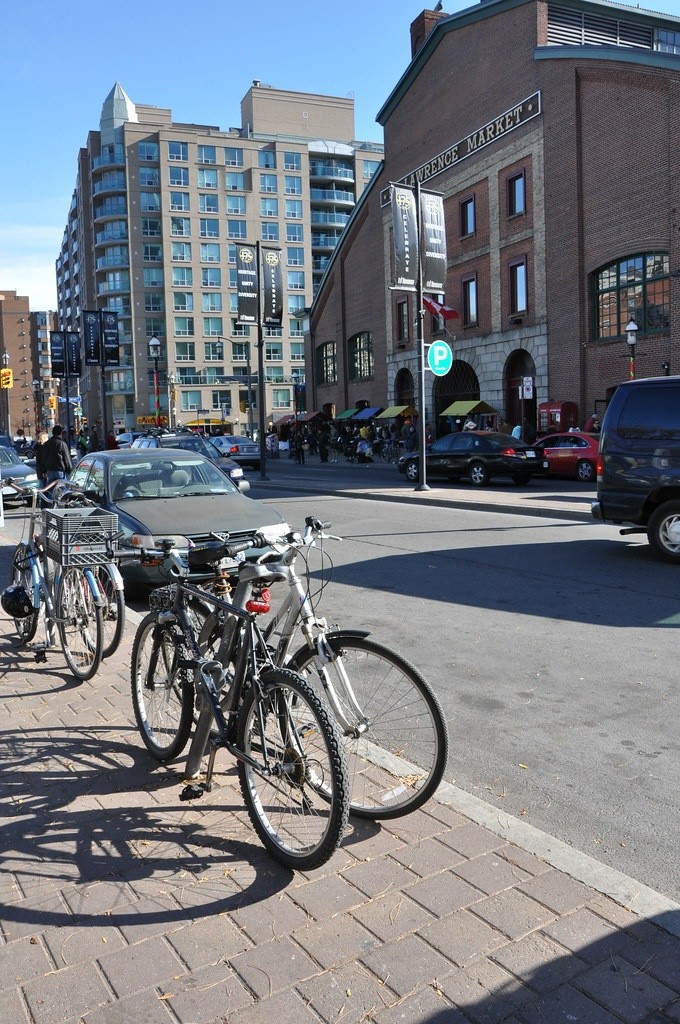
[0,444,41,502]
[399,431,552,487]
[63,448,292,586]
[529,431,602,479]
[116,431,144,449]
[199,436,267,469]
[0,435,38,459]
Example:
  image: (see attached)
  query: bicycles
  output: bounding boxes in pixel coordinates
[0,475,126,683]
[129,518,450,871]
[379,441,399,465]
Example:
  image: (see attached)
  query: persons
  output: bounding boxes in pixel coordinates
[76,425,120,463]
[583,414,601,432]
[36,425,73,510]
[265,419,433,464]
[462,417,535,445]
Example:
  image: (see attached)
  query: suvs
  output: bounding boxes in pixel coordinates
[131,435,251,494]
[589,374,680,564]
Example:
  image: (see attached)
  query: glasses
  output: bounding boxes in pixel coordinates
[595,423,599,424]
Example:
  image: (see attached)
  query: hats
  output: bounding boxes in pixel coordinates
[52,425,65,436]
[592,414,598,420]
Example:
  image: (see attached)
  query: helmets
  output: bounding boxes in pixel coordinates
[0,586,37,618]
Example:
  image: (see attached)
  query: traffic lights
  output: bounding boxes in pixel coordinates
[48,398,56,409]
[240,402,245,412]
[171,389,176,401]
[82,417,87,425]
[1,369,13,389]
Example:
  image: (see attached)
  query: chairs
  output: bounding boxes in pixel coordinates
[160,468,189,487]
[138,474,162,495]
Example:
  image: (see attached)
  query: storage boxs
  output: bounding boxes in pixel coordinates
[41,506,119,566]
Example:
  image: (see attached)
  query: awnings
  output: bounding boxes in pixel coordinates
[439,401,499,416]
[334,405,419,419]
[273,411,320,424]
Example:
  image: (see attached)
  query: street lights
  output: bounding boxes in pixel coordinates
[627,321,639,381]
[215,336,253,440]
[149,336,162,426]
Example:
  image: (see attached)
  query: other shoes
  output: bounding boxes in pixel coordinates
[335,460,337,463]
[330,459,334,462]
